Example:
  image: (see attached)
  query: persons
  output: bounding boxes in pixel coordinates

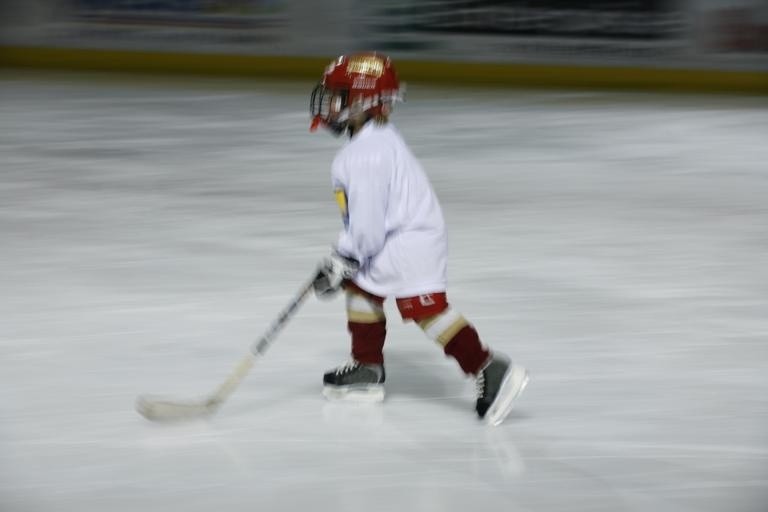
[310,50,512,420]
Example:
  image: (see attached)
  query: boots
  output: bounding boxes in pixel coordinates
[474,355,518,418]
[322,362,387,390]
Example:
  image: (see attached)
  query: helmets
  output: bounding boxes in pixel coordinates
[310,52,405,135]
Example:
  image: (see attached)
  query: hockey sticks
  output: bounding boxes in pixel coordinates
[137,280,315,423]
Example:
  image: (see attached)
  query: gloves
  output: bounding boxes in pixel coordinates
[314,249,361,298]
